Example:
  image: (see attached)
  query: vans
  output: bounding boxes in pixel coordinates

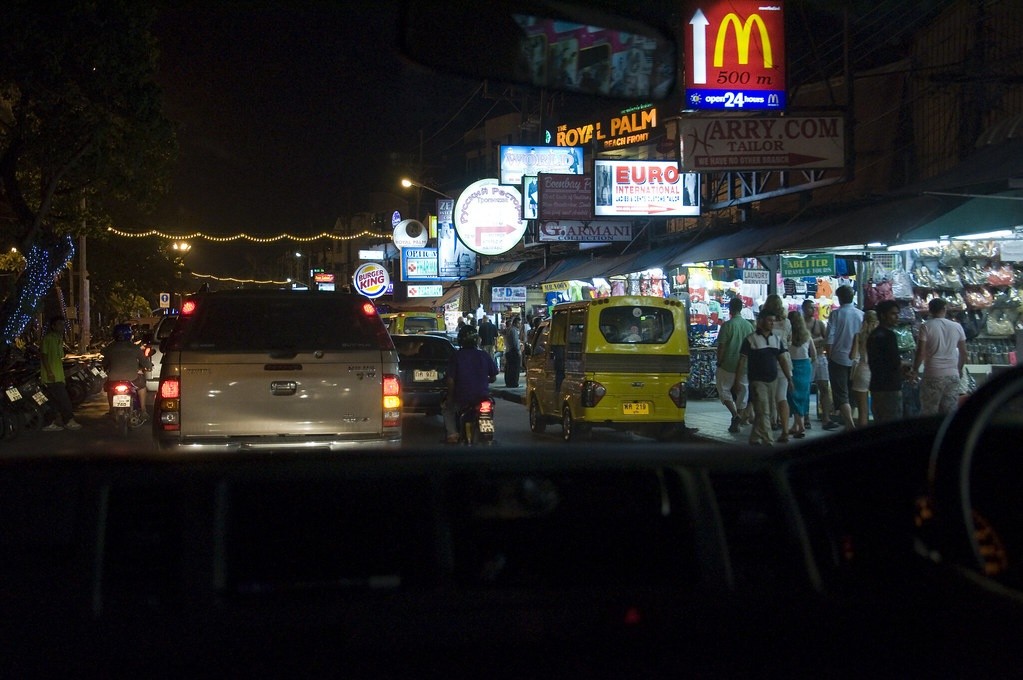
[524,295,692,442]
[143,314,179,392]
[390,334,458,416]
[153,287,405,454]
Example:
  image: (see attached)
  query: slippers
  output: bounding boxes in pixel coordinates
[793,430,806,438]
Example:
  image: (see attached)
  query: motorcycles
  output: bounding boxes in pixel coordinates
[99,346,156,441]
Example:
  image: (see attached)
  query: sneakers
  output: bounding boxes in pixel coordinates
[41,421,64,432]
[63,418,82,431]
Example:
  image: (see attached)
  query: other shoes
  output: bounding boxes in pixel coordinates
[822,421,839,428]
[729,415,742,432]
[440,438,459,444]
[771,422,777,430]
[778,434,789,442]
[805,421,811,428]
[776,420,783,429]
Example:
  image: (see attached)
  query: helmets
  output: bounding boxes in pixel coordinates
[458,325,479,346]
[113,324,132,342]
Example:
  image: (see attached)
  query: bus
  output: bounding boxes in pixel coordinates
[380,311,447,336]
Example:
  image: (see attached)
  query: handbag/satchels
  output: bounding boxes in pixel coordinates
[864,244,1023,351]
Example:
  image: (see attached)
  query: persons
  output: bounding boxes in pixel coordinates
[848,311,879,425]
[716,300,755,434]
[102,323,152,420]
[442,325,499,444]
[866,300,912,420]
[824,285,865,428]
[40,314,83,432]
[788,311,817,439]
[910,298,967,416]
[493,329,504,359]
[456,314,498,359]
[734,310,793,446]
[519,314,541,343]
[801,300,838,429]
[504,317,523,388]
[763,294,793,442]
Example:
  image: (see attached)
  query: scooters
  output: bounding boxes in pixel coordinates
[0,321,152,439]
[435,350,504,447]
